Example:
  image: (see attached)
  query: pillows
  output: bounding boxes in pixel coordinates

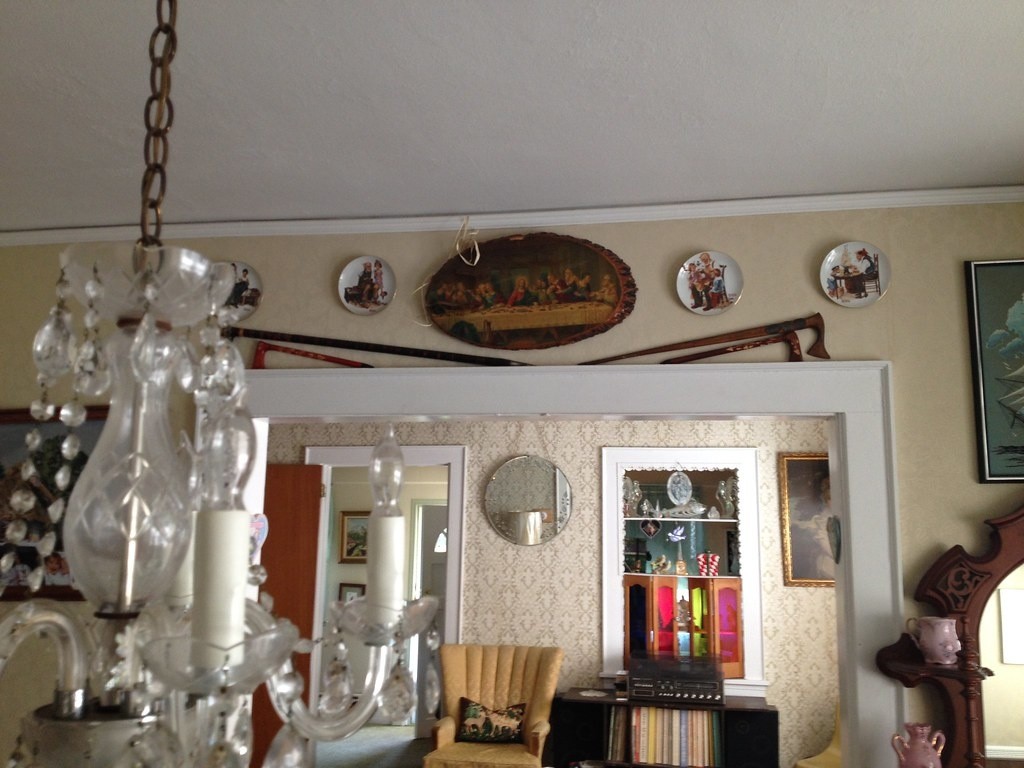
[457,696,527,745]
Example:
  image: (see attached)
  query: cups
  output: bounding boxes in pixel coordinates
[906,616,960,664]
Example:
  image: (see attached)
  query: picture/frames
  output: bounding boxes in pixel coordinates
[966,258,1024,485]
[0,405,111,598]
[778,450,834,586]
[339,583,365,604]
[339,510,370,562]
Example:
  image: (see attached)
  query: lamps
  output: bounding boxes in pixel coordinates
[0,0,425,768]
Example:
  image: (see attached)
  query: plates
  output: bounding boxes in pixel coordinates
[208,261,262,321]
[338,254,397,317]
[676,250,744,315]
[819,242,891,308]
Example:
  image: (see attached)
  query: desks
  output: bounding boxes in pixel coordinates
[568,687,781,768]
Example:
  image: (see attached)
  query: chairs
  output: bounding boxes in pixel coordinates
[426,646,565,768]
[796,705,842,768]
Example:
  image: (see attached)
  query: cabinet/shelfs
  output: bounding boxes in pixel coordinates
[621,518,742,579]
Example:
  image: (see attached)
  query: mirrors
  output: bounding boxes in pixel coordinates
[196,360,908,768]
[484,455,573,546]
[979,563,1024,759]
